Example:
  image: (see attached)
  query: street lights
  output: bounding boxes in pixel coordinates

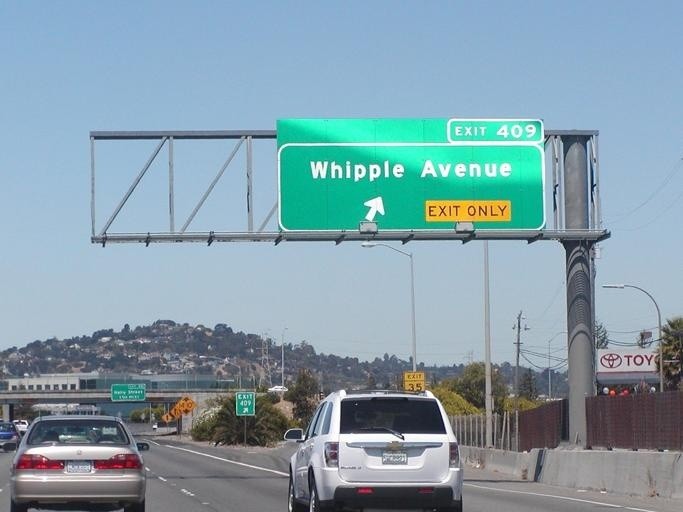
[600,281,665,396]
[15,370,29,391]
[199,354,243,392]
[280,326,290,391]
[161,363,187,390]
[358,240,420,373]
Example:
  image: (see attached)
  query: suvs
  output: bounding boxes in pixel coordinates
[282,386,466,512]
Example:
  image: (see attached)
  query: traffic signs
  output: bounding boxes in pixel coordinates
[236,392,256,417]
[111,383,145,402]
[274,117,546,235]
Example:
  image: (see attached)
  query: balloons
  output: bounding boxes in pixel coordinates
[602,386,657,396]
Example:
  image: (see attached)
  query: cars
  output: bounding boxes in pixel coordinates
[3,412,152,512]
[0,418,32,455]
[267,385,290,394]
